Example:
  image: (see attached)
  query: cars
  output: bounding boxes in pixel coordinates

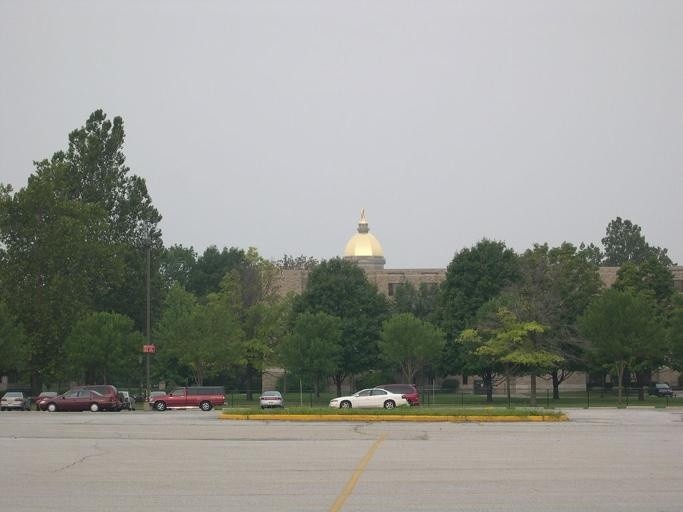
[0,391,30,411]
[78,384,135,412]
[328,387,407,408]
[259,390,284,408]
[648,382,672,397]
[35,392,58,411]
[368,384,419,406]
[39,389,113,412]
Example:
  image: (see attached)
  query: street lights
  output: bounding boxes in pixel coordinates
[142,238,153,400]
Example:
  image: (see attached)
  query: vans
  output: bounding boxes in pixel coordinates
[149,386,226,412]
